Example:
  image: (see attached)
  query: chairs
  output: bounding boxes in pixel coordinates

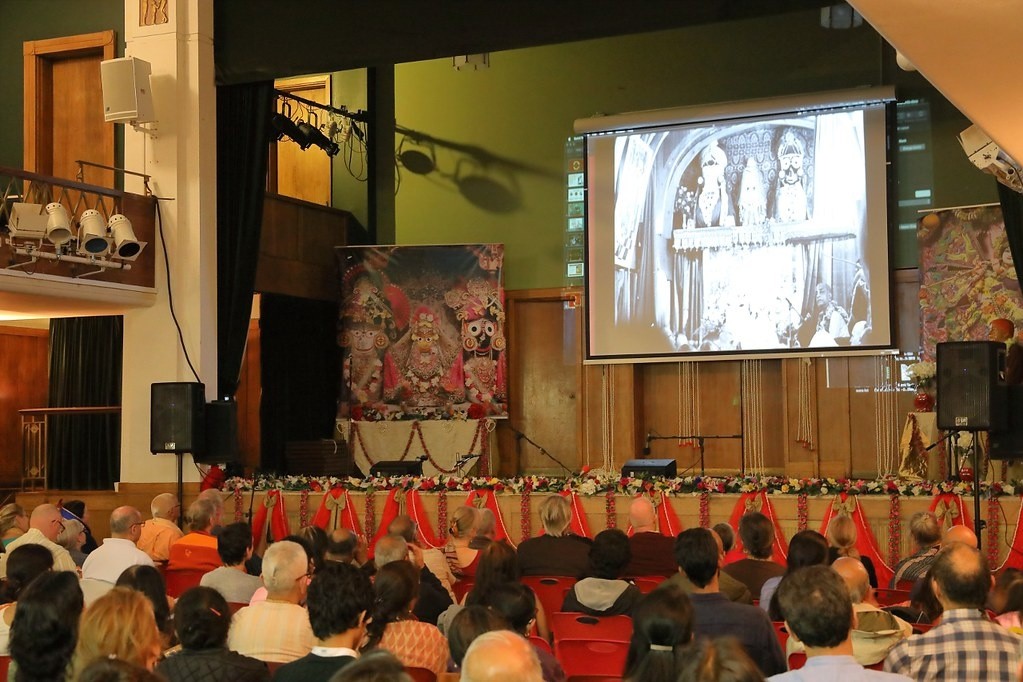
[518,572,668,682]
[162,562,218,601]
[753,587,936,671]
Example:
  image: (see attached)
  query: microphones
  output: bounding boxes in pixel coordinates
[644,433,651,455]
[417,455,428,460]
[462,453,482,458]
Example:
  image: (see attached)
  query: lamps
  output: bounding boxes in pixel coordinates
[275,96,341,160]
[3,201,147,262]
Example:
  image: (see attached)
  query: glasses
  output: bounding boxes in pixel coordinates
[129,520,145,527]
[52,519,66,534]
[526,608,538,625]
[296,572,311,581]
[79,528,87,536]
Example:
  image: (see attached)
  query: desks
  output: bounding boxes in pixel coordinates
[337,419,499,476]
[18,406,121,491]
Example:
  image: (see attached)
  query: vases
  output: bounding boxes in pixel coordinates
[959,468,974,483]
[914,393,935,412]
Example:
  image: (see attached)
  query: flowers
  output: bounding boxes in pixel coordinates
[949,430,974,459]
[905,362,937,393]
[220,466,1023,499]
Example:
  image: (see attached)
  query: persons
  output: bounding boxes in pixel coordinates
[0,491,1023,682]
[989,318,1023,384]
[617,282,873,351]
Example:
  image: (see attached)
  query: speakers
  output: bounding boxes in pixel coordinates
[371,460,422,478]
[621,458,677,492]
[150,382,206,454]
[101,56,155,126]
[194,401,240,463]
[936,340,1008,431]
[989,380,1023,463]
